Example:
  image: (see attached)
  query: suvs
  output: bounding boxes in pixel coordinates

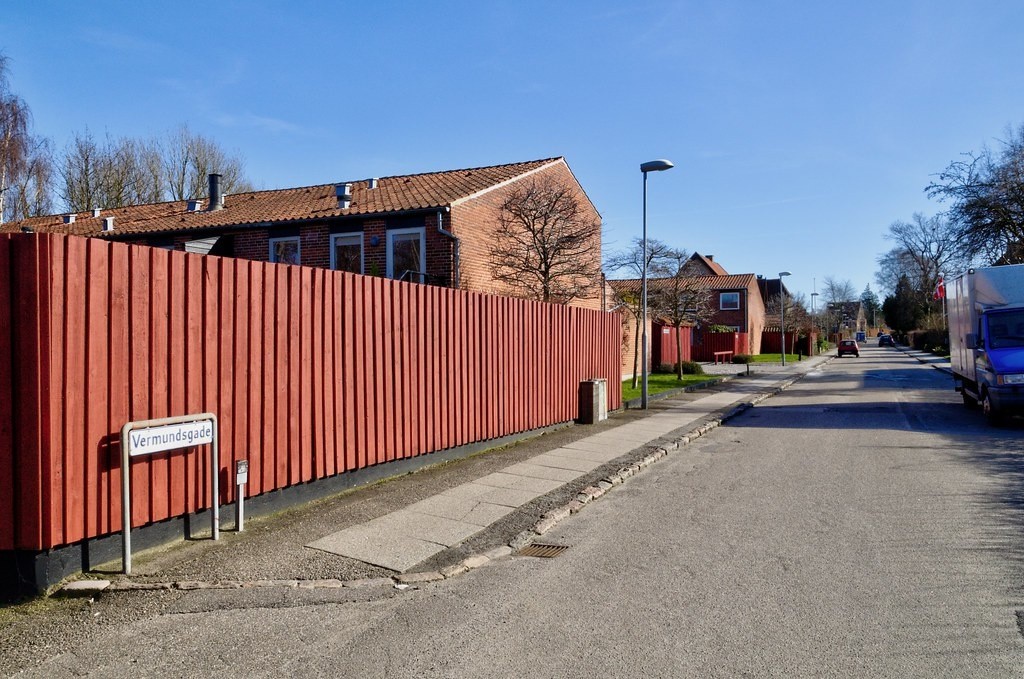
[838,339,859,357]
[879,335,896,347]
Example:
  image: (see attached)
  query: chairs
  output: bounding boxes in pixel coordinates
[993,324,1008,336]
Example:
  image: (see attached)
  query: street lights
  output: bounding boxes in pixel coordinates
[873,309,879,331]
[826,303,834,351]
[778,271,792,367]
[841,314,847,333]
[834,309,841,344]
[640,158,674,410]
[916,289,930,329]
[811,293,819,357]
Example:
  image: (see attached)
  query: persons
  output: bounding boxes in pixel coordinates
[897,334,908,346]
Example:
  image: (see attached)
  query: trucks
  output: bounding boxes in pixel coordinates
[941,263,1024,424]
[855,331,867,343]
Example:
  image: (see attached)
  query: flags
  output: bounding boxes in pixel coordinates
[933,277,945,301]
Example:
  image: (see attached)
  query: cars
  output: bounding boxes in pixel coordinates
[877,332,884,337]
